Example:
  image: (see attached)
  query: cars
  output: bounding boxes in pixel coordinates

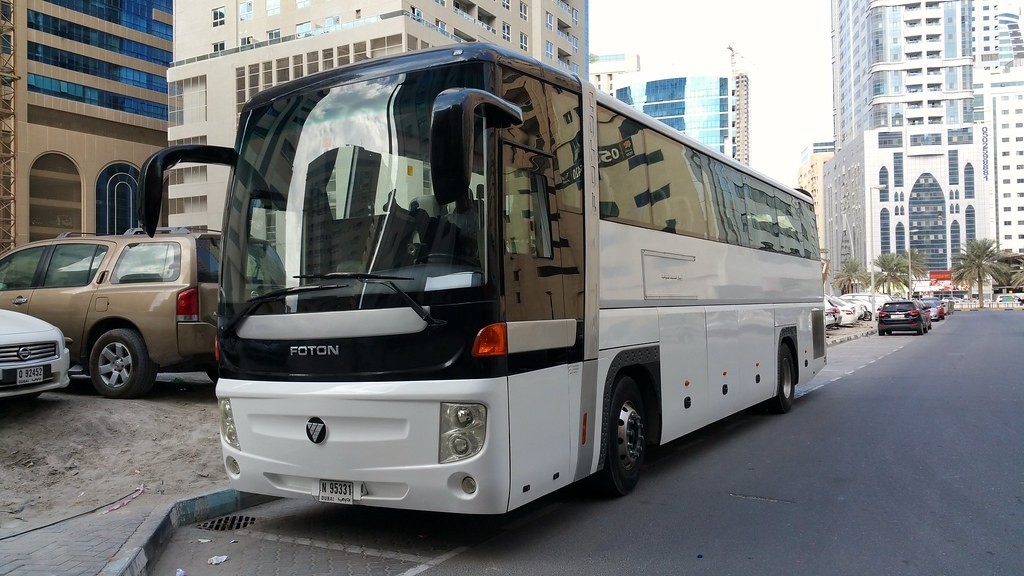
[826,291,1024,337]
[0,309,71,407]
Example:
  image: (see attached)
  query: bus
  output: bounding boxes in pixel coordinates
[137,45,825,526]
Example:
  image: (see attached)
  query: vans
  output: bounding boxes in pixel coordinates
[1,230,282,397]
